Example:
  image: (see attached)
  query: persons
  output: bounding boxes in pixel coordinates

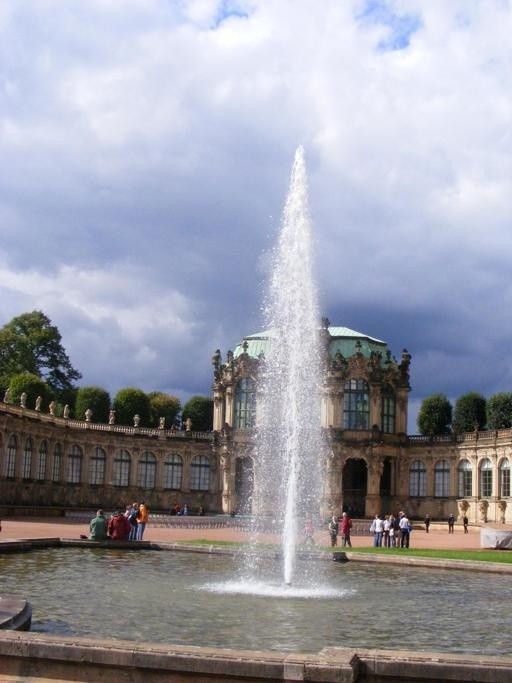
[339,512,353,547]
[300,510,317,546]
[370,510,412,548]
[198,503,206,517]
[463,513,470,534]
[423,514,431,534]
[169,503,190,516]
[88,500,149,542]
[447,514,456,534]
[327,515,339,548]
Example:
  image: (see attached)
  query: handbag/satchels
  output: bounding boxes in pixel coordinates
[389,528,395,536]
[128,515,135,524]
